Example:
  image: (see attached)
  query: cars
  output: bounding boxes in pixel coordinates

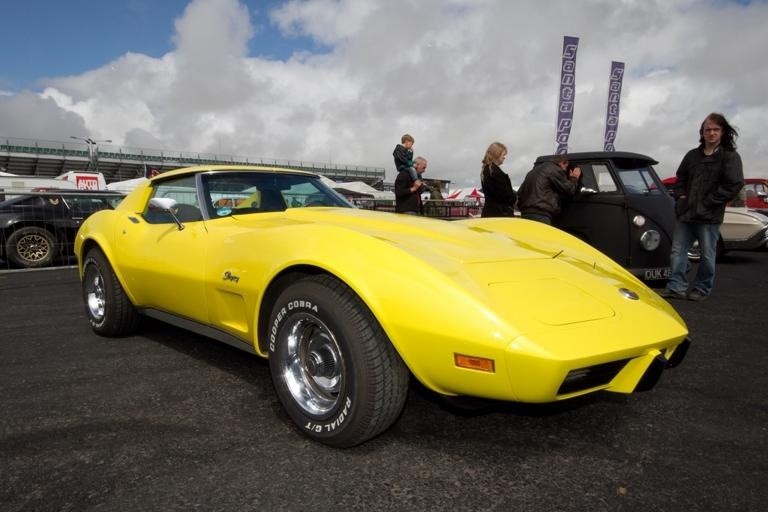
[663,192,768,264]
[526,150,697,286]
[0,188,187,269]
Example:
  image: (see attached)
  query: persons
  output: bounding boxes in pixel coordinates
[427,179,446,217]
[394,156,428,217]
[479,141,518,218]
[392,133,432,194]
[515,154,584,227]
[657,111,745,303]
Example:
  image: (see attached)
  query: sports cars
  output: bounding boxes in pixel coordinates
[69,162,692,451]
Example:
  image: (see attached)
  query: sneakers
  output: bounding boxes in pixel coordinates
[417,183,427,194]
[689,290,710,300]
[658,288,687,298]
